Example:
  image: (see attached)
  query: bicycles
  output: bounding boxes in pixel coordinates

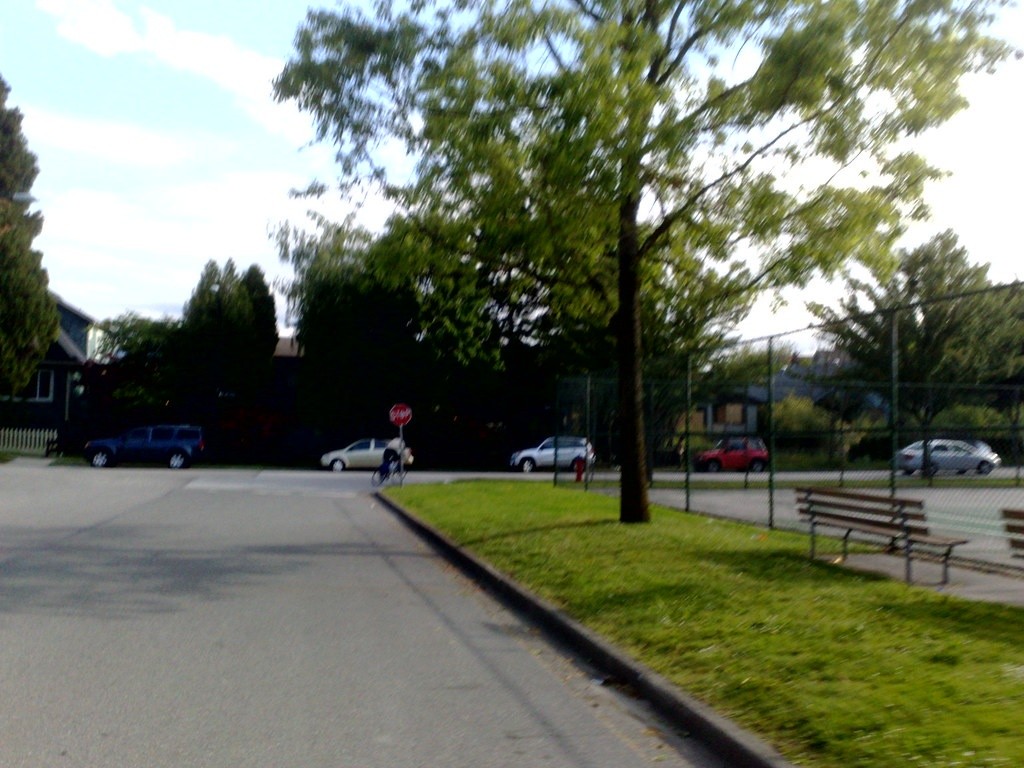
[370,457,410,487]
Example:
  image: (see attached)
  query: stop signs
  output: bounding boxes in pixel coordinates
[389,403,412,427]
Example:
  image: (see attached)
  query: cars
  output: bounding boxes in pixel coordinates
[898,438,1001,479]
[319,438,415,472]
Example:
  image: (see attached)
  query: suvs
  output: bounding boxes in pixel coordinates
[694,436,769,473]
[84,425,206,470]
[509,435,597,474]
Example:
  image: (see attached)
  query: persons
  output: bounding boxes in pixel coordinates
[383,437,405,471]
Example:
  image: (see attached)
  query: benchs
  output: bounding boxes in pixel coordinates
[793,483,971,587]
[1002,506,1023,563]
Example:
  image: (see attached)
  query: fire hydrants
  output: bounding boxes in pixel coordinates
[575,457,586,483]
